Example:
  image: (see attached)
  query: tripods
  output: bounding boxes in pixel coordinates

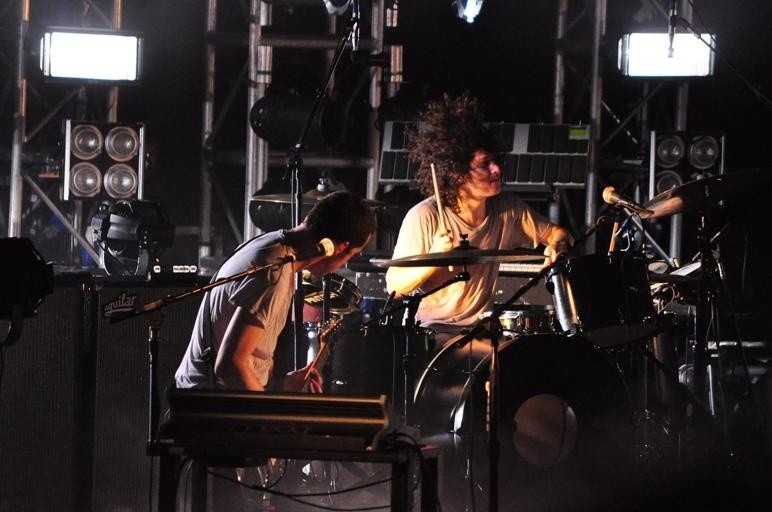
[694,251,754,398]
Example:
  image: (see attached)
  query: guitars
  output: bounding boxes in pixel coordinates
[236,316,346,512]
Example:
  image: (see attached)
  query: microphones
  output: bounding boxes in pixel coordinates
[603,185,655,215]
[277,238,335,267]
[351,0,361,51]
[668,0,677,35]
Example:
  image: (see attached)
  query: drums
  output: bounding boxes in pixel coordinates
[321,296,416,426]
[413,328,641,512]
[300,267,362,315]
[545,251,668,348]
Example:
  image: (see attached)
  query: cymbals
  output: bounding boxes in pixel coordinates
[249,188,388,210]
[639,172,727,219]
[386,246,554,267]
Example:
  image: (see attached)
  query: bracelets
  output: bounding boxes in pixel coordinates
[266,367,288,392]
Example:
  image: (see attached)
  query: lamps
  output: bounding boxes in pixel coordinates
[33,25,146,87]
[616,29,718,79]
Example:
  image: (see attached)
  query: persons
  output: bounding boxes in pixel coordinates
[171,184,423,511]
[382,88,578,379]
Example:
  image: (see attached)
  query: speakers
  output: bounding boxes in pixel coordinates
[648,130,725,209]
[61,118,148,202]
